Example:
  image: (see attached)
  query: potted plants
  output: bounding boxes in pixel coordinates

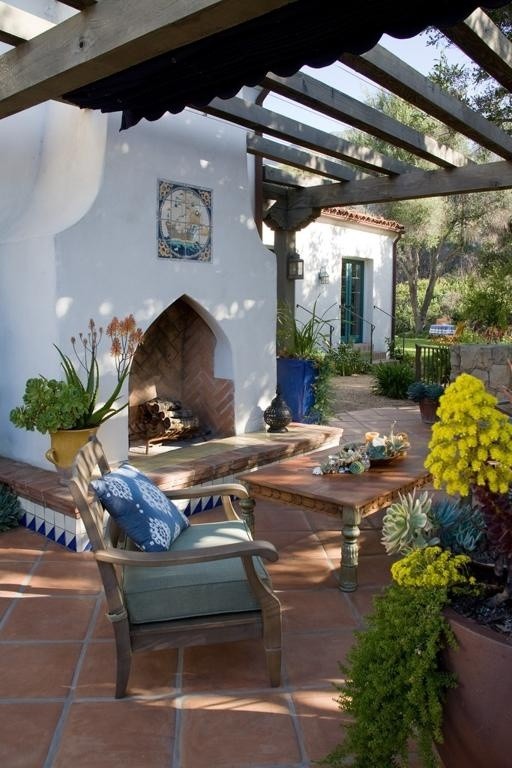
[334,603,512,768]
[276,292,357,425]
[407,382,445,424]
[7,312,145,487]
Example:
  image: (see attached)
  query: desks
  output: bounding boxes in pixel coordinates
[428,325,456,336]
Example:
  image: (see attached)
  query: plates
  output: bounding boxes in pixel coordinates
[342,441,401,467]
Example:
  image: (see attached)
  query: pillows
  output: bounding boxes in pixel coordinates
[90,459,191,552]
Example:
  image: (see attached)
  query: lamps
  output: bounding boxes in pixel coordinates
[286,252,304,280]
[318,266,330,285]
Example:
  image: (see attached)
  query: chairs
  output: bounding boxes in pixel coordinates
[64,432,286,698]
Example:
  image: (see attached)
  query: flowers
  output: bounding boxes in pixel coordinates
[377,373,512,590]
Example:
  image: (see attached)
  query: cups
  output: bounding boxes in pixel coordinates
[364,432,379,442]
